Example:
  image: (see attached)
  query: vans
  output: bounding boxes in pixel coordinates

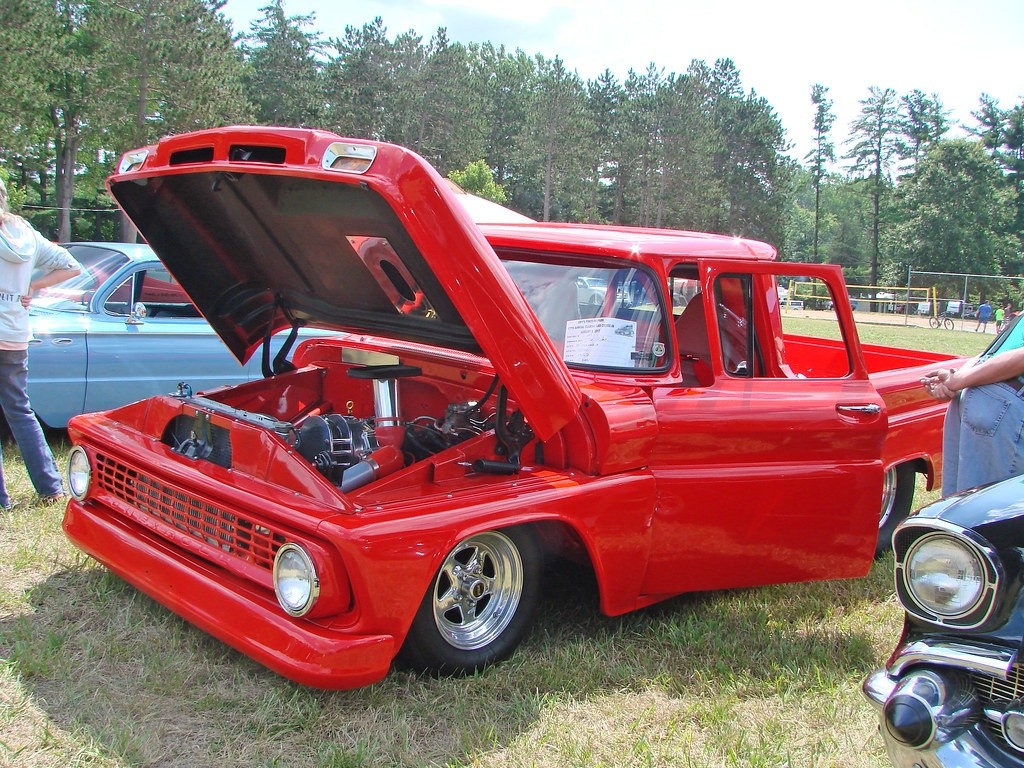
[779,300,804,310]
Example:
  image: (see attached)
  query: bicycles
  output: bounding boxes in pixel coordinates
[929,312,954,331]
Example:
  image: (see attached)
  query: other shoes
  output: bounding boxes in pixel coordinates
[42,490,68,502]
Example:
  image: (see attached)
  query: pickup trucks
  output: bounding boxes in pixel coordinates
[60,125,977,691]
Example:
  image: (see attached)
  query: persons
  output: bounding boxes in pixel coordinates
[0,178,81,512]
[975,300,992,333]
[919,309,1024,499]
[995,303,1012,333]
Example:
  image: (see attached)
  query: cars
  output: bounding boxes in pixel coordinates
[577,276,631,309]
[0,242,343,442]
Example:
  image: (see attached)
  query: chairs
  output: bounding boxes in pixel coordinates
[664,289,733,388]
[542,280,581,338]
